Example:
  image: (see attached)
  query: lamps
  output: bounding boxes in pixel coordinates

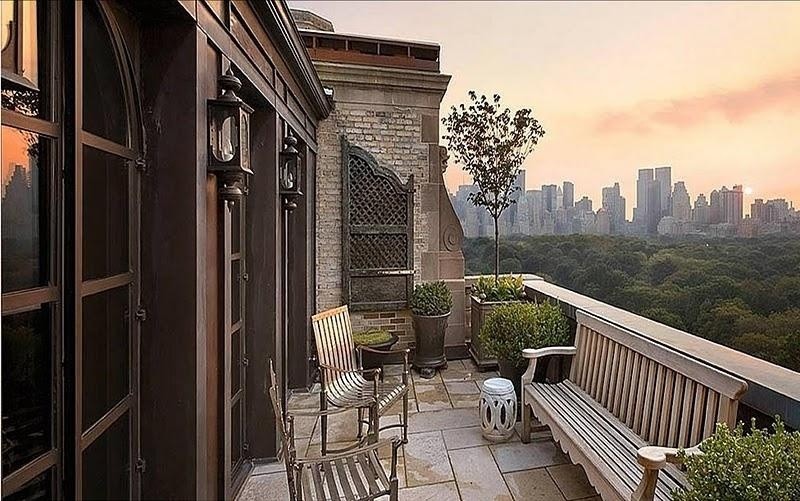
[311,304,411,456]
[269,357,398,501]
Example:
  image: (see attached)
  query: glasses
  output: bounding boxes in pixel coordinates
[279,128,309,214]
[208,65,256,212]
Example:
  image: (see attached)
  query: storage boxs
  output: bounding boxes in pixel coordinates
[520,309,749,501]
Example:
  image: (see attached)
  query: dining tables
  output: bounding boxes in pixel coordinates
[441,91,545,373]
[349,327,399,384]
[477,295,572,422]
[409,279,454,379]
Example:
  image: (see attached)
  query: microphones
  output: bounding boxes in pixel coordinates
[478,377,518,444]
